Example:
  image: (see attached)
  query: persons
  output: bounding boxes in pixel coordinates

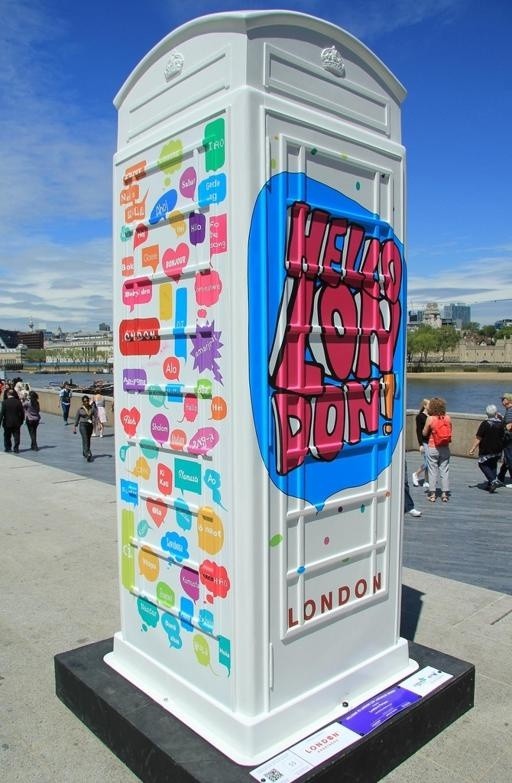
[405,455,422,516]
[413,398,431,487]
[0,377,40,452]
[58,382,72,424]
[469,403,504,492]
[422,396,452,501]
[496,393,512,488]
[74,395,104,461]
[92,394,107,437]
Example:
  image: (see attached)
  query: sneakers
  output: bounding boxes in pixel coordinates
[490,480,503,492]
[409,509,422,517]
[412,472,419,486]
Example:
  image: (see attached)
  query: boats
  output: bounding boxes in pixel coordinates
[43,378,113,399]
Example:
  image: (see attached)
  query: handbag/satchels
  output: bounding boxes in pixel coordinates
[432,417,452,446]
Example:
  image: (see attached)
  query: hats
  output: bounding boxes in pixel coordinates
[500,393,512,400]
